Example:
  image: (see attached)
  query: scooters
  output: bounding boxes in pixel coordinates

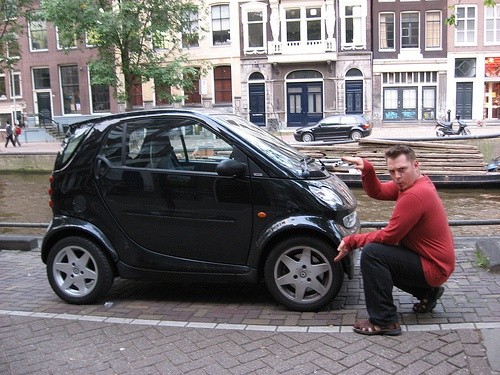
[434,114,471,137]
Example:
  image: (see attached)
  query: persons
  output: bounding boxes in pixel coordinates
[334,145,455,336]
[5,120,21,148]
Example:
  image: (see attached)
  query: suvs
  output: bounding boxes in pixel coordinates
[293,114,374,143]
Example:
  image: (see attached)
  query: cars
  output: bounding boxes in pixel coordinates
[40,107,361,312]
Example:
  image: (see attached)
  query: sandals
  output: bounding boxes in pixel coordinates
[353,319,402,335]
[414,287,444,312]
